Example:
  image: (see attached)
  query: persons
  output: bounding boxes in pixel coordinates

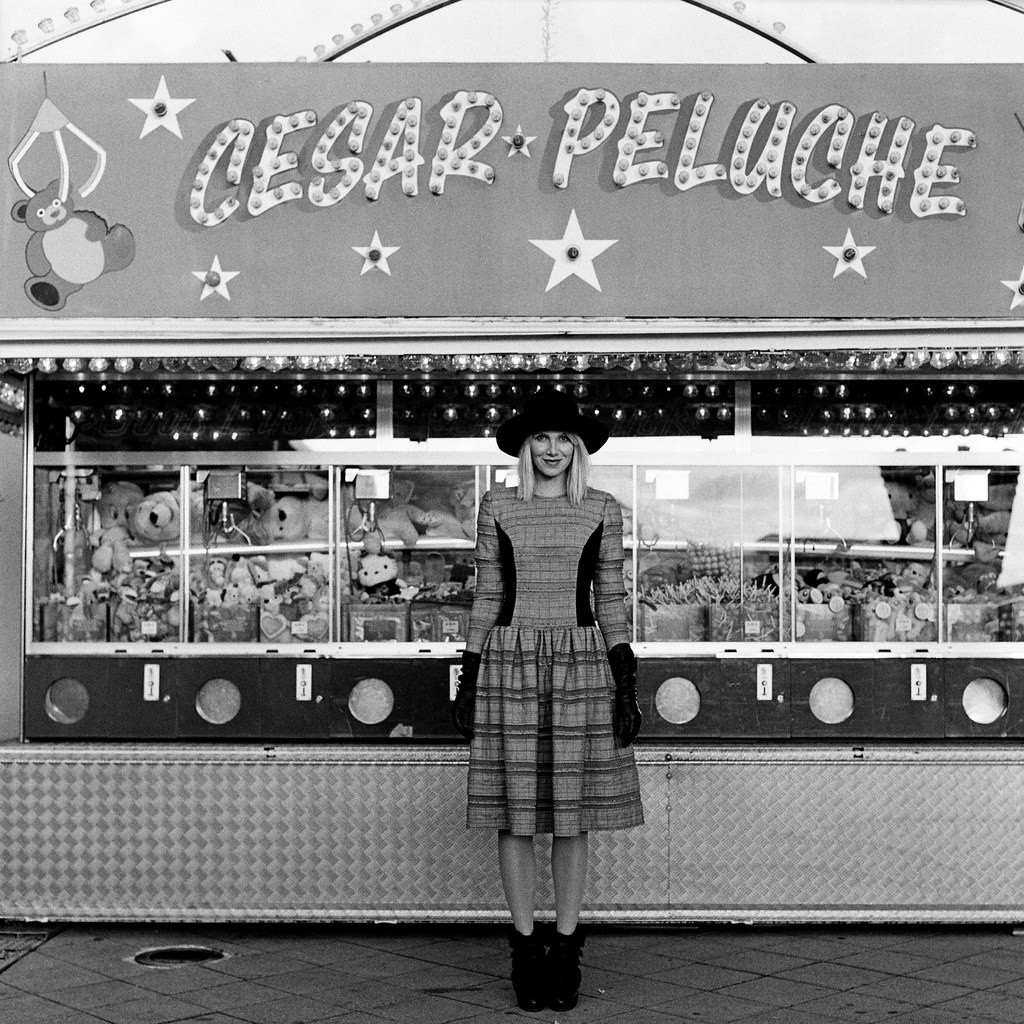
[450,389,645,1012]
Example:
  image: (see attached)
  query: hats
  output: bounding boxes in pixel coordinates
[495,389,609,458]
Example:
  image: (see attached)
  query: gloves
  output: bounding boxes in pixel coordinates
[607,642,643,748]
[451,650,480,739]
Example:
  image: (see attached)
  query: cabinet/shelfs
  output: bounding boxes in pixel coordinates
[21,371,1024,744]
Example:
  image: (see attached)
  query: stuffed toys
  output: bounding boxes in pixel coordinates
[611,467,1024,643]
[56,467,474,642]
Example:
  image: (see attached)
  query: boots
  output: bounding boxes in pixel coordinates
[546,920,587,1011]
[506,922,547,1012]
